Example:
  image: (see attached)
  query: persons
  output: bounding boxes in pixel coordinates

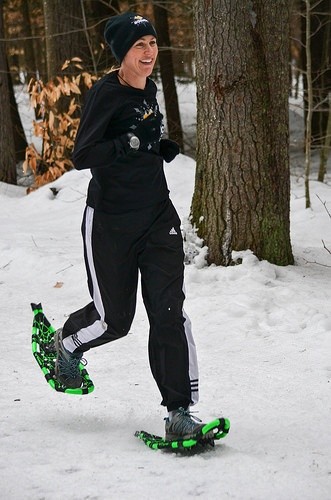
[53,12,214,442]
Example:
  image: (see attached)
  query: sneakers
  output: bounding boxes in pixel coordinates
[164,410,213,440]
[54,327,88,389]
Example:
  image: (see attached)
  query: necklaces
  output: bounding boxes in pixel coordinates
[117,72,146,89]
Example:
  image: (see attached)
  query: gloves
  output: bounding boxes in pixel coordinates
[159,139,180,162]
[118,109,163,155]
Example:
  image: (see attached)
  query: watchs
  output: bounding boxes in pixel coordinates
[126,132,140,150]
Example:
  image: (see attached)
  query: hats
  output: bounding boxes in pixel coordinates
[104,12,159,65]
